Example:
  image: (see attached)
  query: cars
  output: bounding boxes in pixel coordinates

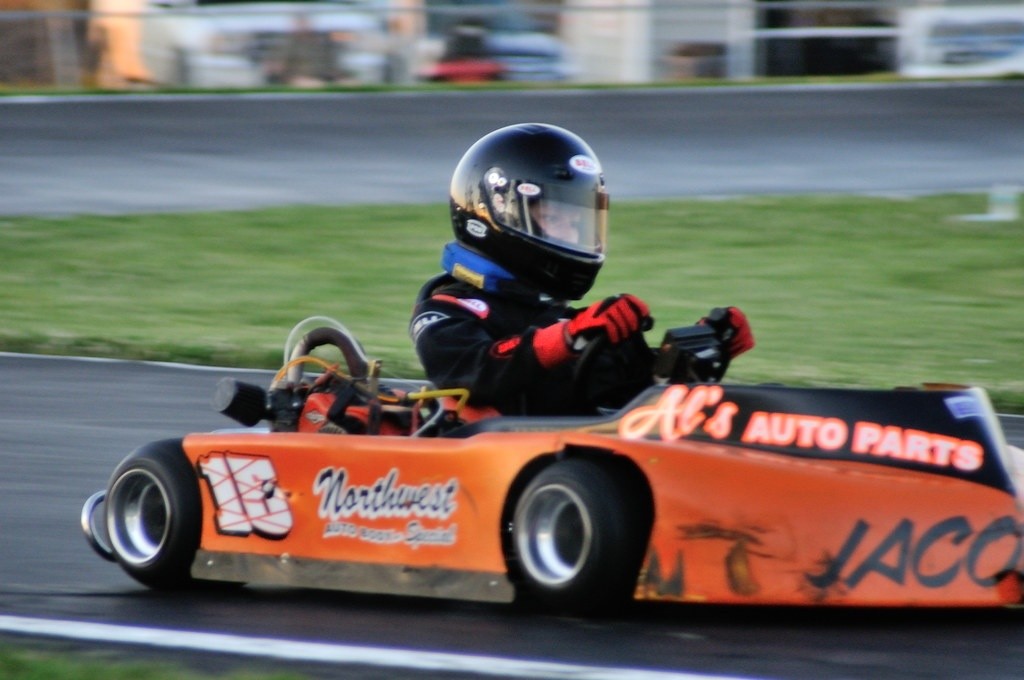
[411,30,580,86]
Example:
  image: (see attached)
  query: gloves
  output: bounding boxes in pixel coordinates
[564,292,649,347]
[694,306,755,360]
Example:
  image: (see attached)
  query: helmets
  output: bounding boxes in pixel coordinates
[449,120,612,309]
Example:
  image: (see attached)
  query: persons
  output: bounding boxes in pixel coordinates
[406,121,754,417]
